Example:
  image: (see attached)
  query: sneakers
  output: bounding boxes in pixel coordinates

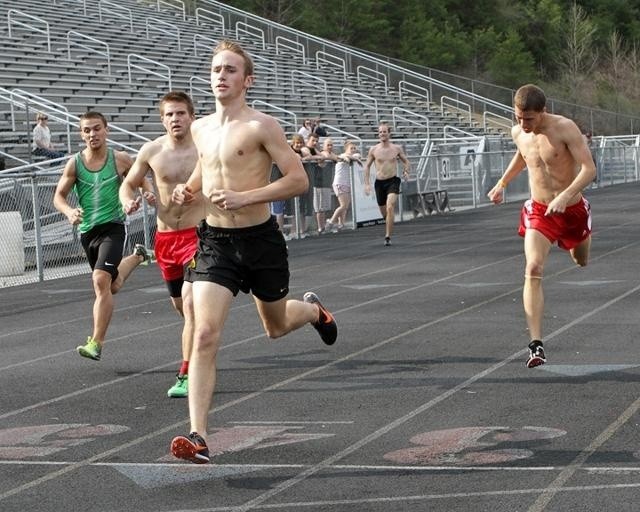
[525,340,548,369]
[302,290,339,346]
[166,372,188,398]
[383,236,392,247]
[134,242,155,267]
[281,218,353,241]
[76,334,103,361]
[170,429,210,464]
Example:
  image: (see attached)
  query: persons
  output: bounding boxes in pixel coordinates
[363,124,410,246]
[117,92,202,397]
[170,42,338,466]
[272,116,363,242]
[31,113,64,168]
[51,111,159,361]
[486,84,597,368]
[585,132,600,191]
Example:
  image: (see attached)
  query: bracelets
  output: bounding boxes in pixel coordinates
[403,170,409,173]
[498,180,506,188]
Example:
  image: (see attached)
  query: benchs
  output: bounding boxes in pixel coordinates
[407,189,456,219]
